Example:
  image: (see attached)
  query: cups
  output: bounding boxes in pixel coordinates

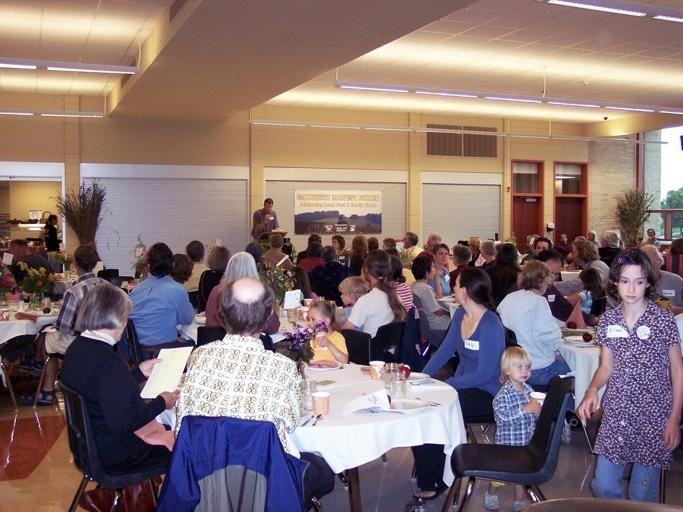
[315,334,324,348]
[64,271,70,281]
[529,391,546,406]
[368,360,406,401]
[286,309,299,323]
[303,299,313,307]
[41,297,50,313]
[0,309,9,321]
[300,378,330,417]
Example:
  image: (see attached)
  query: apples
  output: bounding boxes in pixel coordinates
[583,333,592,342]
[400,365,411,378]
[567,321,577,329]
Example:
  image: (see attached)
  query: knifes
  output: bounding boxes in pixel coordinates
[301,414,314,427]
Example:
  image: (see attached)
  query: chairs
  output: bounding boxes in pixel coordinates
[0,273,681,512]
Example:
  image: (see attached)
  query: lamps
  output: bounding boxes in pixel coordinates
[0,37,141,75]
[0,90,107,121]
[549,0,682,23]
[250,114,671,144]
[336,65,682,117]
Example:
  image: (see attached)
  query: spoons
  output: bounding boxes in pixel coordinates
[312,414,322,426]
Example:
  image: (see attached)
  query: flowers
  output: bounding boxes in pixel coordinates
[14,259,60,295]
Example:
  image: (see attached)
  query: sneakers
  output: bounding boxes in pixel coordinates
[510,499,529,512]
[484,488,501,511]
[19,354,47,376]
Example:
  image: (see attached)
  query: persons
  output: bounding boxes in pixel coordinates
[45,215,62,251]
[251,198,280,240]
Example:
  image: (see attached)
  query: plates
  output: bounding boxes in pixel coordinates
[563,327,587,331]
[37,311,57,316]
[564,335,595,346]
[368,398,429,414]
[404,370,430,383]
[192,317,205,324]
[305,361,343,370]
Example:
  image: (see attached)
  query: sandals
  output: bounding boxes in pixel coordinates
[20,386,57,405]
[413,481,449,500]
